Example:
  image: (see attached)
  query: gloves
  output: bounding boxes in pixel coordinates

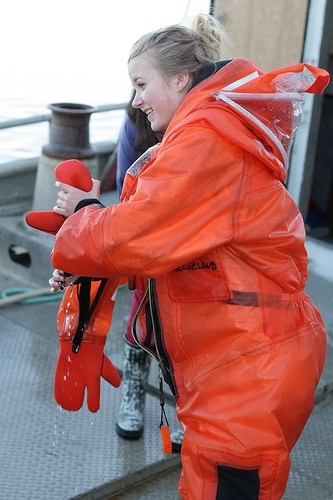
[25,159,92,235]
[53,340,121,413]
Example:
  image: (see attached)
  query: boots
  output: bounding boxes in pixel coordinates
[115,342,152,438]
[169,427,184,453]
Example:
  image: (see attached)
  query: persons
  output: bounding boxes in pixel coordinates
[117,89,167,442]
[48,12,329,499]
